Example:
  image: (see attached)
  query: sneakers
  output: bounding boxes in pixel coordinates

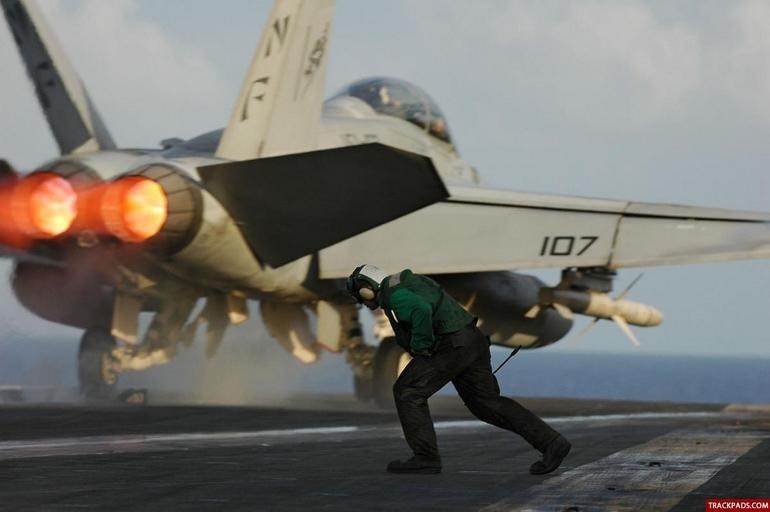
[388,458,442,474]
[529,434,571,475]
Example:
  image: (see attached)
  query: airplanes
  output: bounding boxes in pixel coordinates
[0,0,770,409]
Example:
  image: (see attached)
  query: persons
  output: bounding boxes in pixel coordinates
[346,262,573,476]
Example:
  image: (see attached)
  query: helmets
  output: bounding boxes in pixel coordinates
[346,264,387,310]
